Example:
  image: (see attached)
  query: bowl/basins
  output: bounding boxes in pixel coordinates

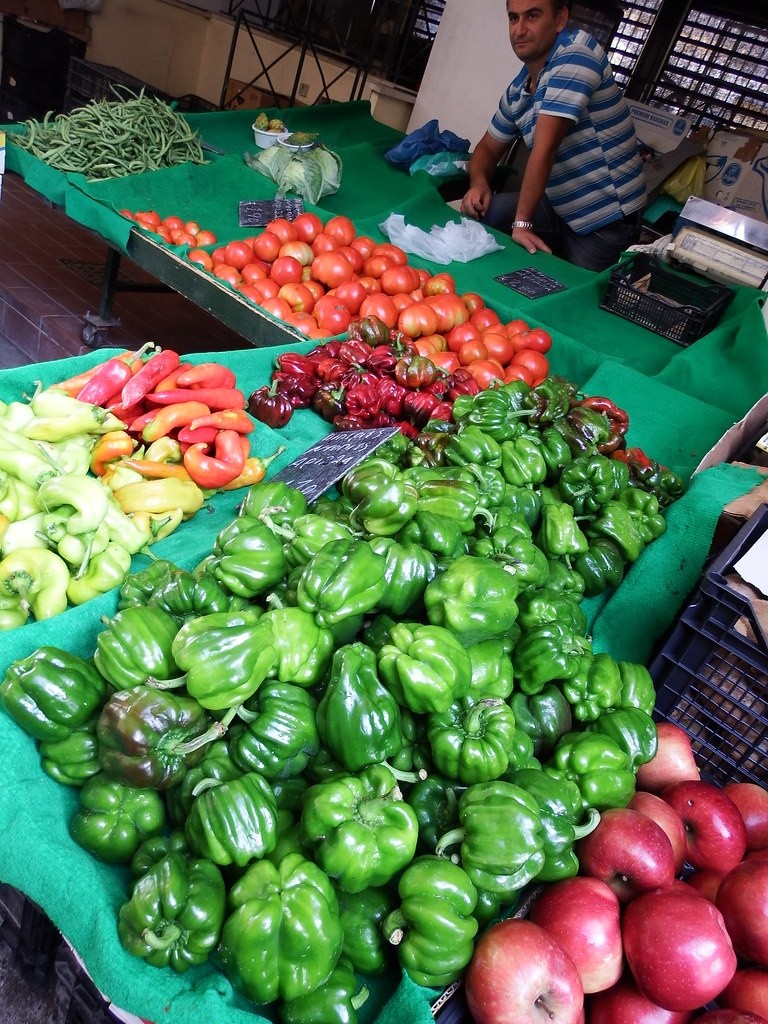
[252,123,289,149]
[277,133,314,152]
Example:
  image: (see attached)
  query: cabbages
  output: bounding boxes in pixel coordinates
[243,146,343,206]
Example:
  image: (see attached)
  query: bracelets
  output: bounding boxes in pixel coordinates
[511,220,532,229]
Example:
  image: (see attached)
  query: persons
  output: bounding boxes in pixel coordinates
[460,0,649,272]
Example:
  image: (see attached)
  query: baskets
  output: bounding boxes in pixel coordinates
[646,502,768,791]
[598,251,735,347]
[62,55,224,120]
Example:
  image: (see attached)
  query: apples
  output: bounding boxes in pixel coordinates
[470,724,768,1024]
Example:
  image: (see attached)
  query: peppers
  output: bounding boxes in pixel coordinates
[0,316,682,1024]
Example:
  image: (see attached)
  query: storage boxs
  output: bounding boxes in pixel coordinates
[1,1,228,125]
[599,252,736,349]
[0,881,124,1024]
[648,392,768,793]
[226,77,309,110]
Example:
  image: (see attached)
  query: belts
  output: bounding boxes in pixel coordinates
[621,212,639,225]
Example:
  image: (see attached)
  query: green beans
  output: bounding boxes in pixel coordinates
[8,84,212,181]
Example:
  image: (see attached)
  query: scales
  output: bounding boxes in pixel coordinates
[667,195,768,289]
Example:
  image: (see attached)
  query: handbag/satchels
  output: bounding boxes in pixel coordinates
[409,151,472,178]
[662,154,707,205]
[385,119,471,169]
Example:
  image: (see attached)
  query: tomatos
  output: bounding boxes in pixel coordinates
[125,210,547,389]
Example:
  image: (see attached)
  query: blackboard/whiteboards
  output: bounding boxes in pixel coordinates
[492,266,569,301]
[267,426,403,507]
[238,199,304,227]
